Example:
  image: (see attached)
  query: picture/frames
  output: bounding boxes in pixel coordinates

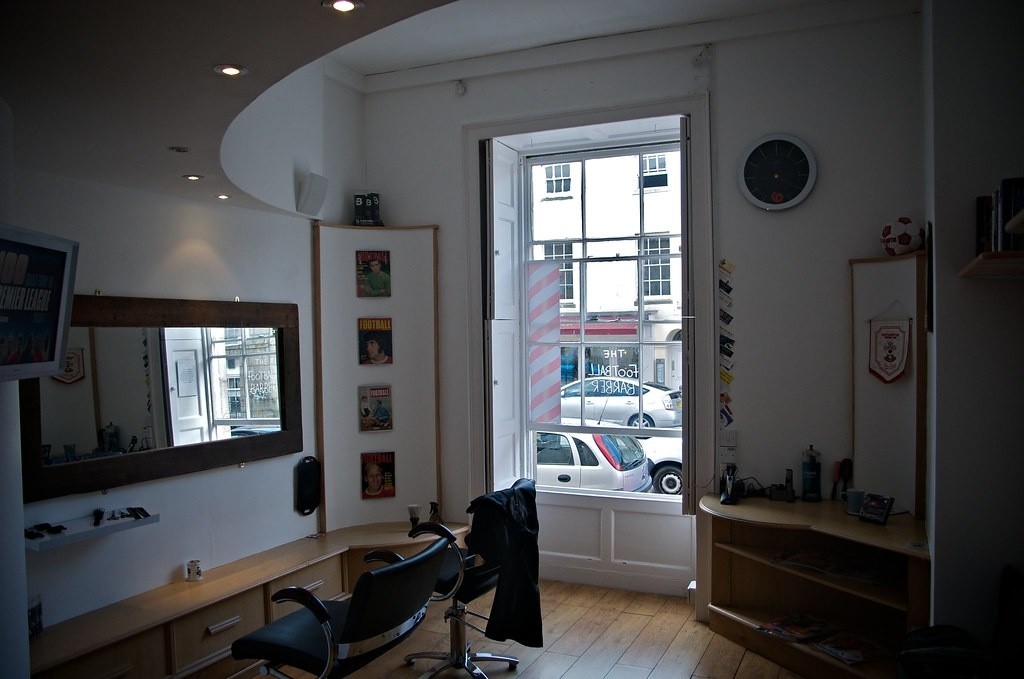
[0,222,80,384]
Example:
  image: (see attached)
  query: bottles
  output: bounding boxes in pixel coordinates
[801,444,822,503]
[104,422,118,452]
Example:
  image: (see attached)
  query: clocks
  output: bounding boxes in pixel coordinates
[739,136,819,211]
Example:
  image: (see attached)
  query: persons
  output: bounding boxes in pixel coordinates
[361,332,393,364]
[363,258,391,297]
[372,400,389,428]
[361,396,371,423]
[362,462,395,498]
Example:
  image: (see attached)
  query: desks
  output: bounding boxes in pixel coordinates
[31,520,469,679]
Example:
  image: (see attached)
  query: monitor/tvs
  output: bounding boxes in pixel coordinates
[0,222,80,381]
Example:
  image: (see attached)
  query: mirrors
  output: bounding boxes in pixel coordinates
[19,293,303,504]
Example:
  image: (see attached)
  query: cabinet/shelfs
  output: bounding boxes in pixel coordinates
[699,491,933,679]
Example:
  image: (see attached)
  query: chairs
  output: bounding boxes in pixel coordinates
[403,478,544,679]
[231,518,463,679]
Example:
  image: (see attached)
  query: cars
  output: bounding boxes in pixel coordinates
[231,426,281,438]
[636,437,684,495]
[535,425,653,492]
[561,376,683,439]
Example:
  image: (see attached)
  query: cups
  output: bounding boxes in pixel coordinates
[64,443,76,459]
[840,489,864,515]
[187,559,202,580]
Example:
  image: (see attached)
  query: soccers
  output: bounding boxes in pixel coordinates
[880,216,925,257]
